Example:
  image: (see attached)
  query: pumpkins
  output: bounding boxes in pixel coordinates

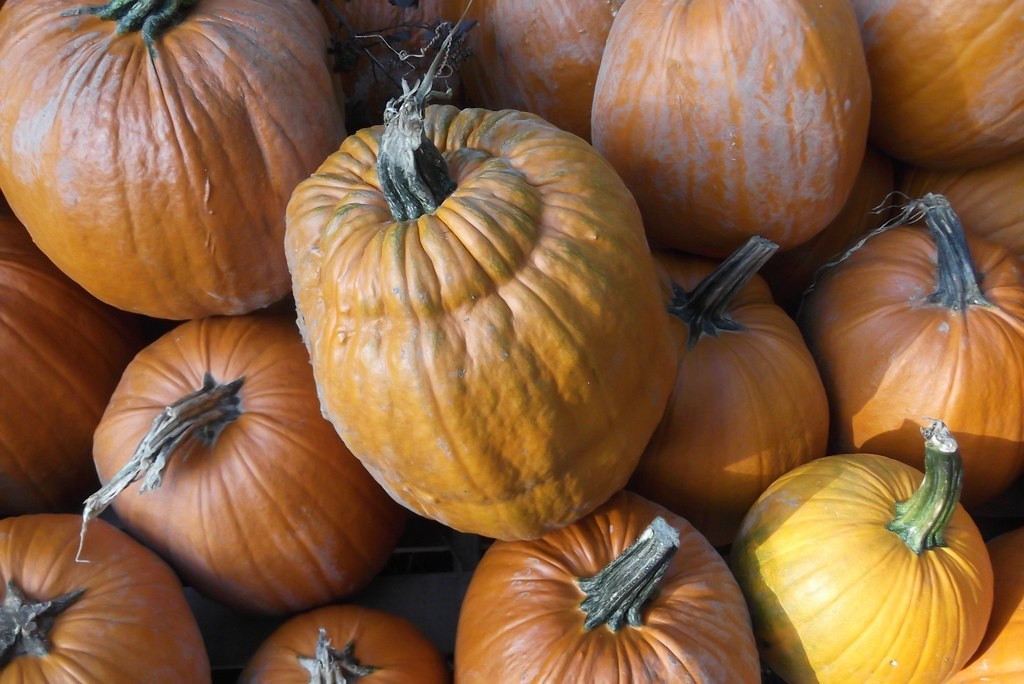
[0,0,1024,684]
